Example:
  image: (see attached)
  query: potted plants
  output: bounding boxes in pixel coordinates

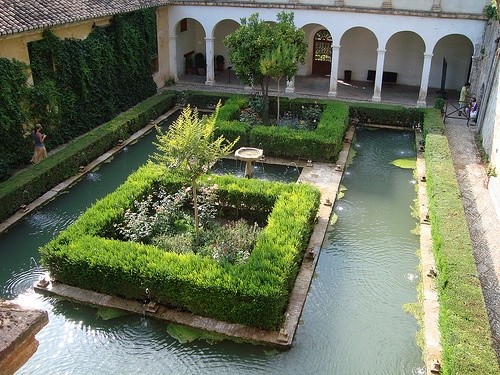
[434,98,445,118]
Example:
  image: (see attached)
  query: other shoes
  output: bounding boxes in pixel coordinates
[458,111,462,117]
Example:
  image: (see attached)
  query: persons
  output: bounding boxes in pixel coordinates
[459,82,471,112]
[30,124,48,164]
[467,107,477,127]
[465,97,478,116]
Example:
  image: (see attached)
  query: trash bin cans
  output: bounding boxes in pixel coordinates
[344,70,351,82]
